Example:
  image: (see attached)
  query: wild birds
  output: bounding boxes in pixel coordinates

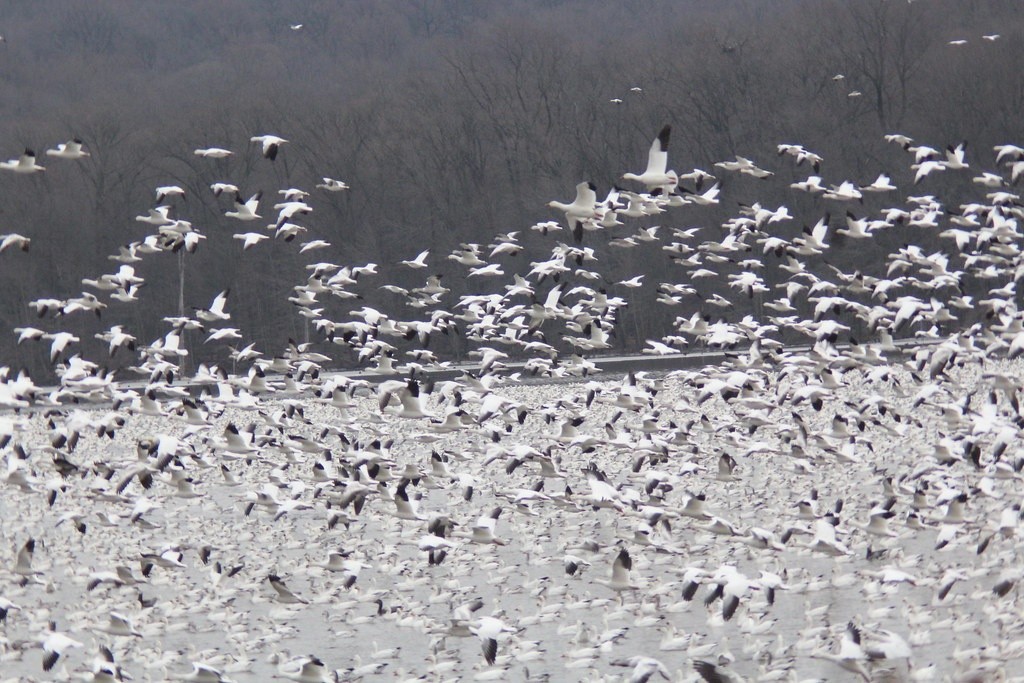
[949,39,968,46]
[288,24,303,31]
[832,74,845,81]
[610,98,623,104]
[848,90,862,97]
[630,87,643,92]
[982,34,1001,41]
[1,124,1023,682]
